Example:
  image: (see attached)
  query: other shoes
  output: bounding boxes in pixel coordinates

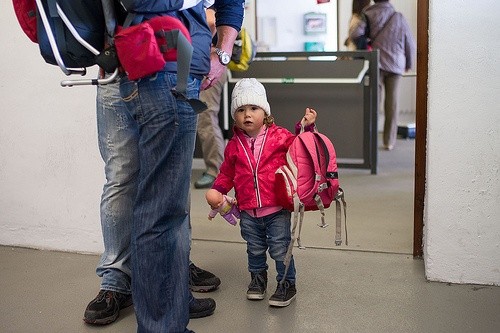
[194,173,215,189]
[384,144,393,150]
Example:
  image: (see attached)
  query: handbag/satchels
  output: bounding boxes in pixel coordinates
[355,35,372,58]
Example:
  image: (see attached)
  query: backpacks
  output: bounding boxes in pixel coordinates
[228,27,254,72]
[275,116,338,211]
[12,0,103,68]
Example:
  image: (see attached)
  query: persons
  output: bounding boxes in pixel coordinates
[206,78,317,306]
[83,0,245,333]
[347,0,414,150]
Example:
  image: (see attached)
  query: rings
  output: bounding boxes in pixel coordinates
[206,77,213,82]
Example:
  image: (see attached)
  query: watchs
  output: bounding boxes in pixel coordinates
[213,48,231,65]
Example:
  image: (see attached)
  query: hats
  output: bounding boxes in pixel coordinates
[231,78,270,120]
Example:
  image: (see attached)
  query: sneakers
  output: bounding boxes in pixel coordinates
[188,258,221,292]
[83,288,131,326]
[269,280,297,306]
[189,292,216,319]
[246,272,267,300]
